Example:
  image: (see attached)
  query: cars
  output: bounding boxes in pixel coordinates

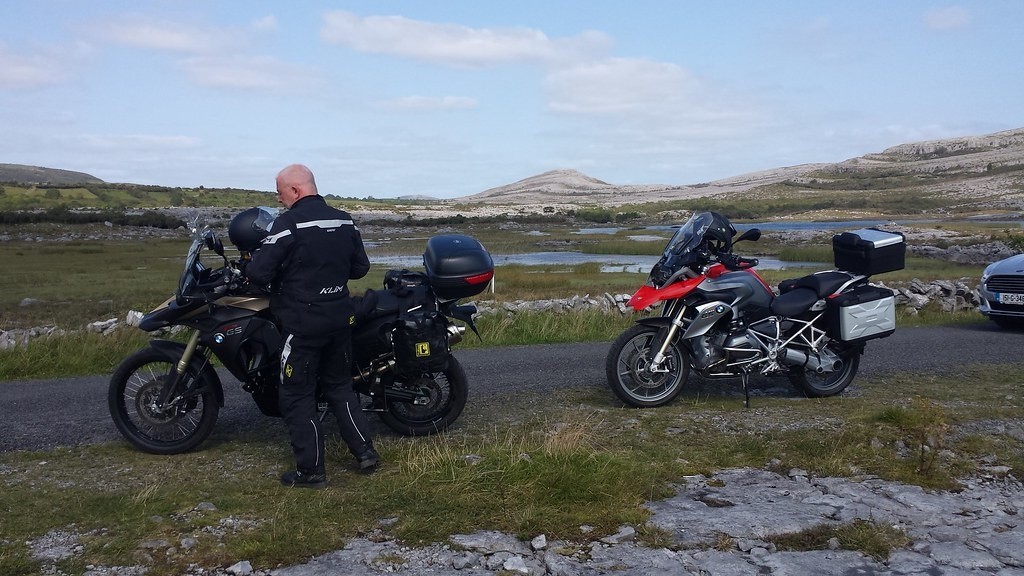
[979,252,1024,332]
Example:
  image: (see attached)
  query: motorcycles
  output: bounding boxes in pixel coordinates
[107,213,498,456]
[604,212,909,408]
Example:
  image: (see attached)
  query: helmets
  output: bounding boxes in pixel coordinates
[228,208,274,253]
[693,212,736,254]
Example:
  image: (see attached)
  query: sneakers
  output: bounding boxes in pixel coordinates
[357,447,382,468]
[281,471,326,488]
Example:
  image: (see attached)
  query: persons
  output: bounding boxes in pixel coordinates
[227,164,382,487]
